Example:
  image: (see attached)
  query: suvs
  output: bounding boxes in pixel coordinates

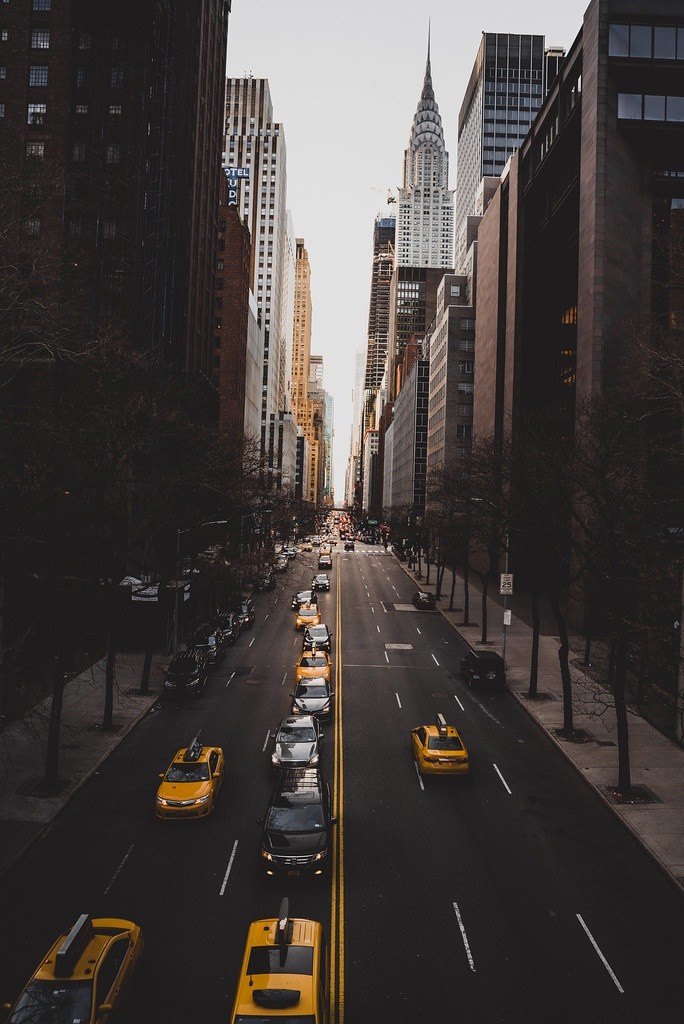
[163,597,256,698]
[257,768,338,880]
[253,569,276,594]
[457,650,507,689]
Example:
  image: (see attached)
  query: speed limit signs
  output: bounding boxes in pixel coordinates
[501,574,514,594]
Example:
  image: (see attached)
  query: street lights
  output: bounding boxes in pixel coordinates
[172,520,227,656]
[469,497,506,668]
[239,510,272,559]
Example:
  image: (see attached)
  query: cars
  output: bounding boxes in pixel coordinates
[273,548,296,573]
[311,573,331,592]
[413,591,436,608]
[270,715,324,768]
[301,511,374,570]
[231,896,323,1024]
[409,714,470,777]
[154,729,224,820]
[289,591,336,722]
[5,914,144,1023]
